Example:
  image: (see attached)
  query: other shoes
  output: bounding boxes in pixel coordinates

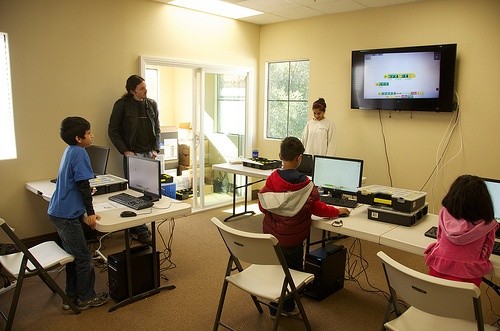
[267,306,276,319]
[130,230,152,246]
[281,303,300,316]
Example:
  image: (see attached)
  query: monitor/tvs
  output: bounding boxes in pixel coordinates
[85,145,110,178]
[312,155,364,198]
[481,178,500,224]
[127,156,161,202]
[351,44,457,112]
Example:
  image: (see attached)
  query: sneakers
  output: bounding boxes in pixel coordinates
[63,294,76,309]
[76,291,110,310]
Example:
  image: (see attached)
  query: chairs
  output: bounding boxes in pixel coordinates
[376,251,498,331]
[0,217,81,331]
[210,217,315,331]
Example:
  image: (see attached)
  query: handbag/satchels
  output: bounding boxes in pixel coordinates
[214,171,229,194]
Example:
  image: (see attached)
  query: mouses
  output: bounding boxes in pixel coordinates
[339,214,348,217]
[120,211,137,217]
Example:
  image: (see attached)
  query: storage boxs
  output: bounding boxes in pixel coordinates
[243,160,282,170]
[88,174,128,196]
[357,185,429,227]
[161,139,220,200]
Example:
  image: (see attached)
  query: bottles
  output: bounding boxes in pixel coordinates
[252,146,258,160]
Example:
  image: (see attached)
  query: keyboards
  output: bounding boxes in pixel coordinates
[320,196,359,208]
[424,226,500,255]
[108,193,154,210]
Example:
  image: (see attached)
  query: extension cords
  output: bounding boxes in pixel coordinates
[95,250,108,263]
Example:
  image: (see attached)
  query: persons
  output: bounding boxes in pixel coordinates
[48,116,110,311]
[423,175,500,288]
[257,137,350,320]
[108,75,161,244]
[298,98,339,163]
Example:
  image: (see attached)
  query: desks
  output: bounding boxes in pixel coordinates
[26,171,192,312]
[300,198,500,302]
[212,159,366,222]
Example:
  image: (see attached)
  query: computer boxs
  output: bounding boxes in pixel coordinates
[304,242,347,301]
[107,244,160,303]
